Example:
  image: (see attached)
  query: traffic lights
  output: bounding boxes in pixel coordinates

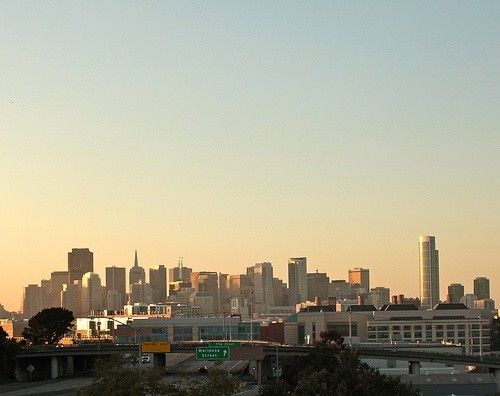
[272,367,275,371]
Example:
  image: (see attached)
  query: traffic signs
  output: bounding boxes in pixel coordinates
[194,346,230,360]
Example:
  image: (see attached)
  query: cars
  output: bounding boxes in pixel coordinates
[131,357,139,363]
[198,366,208,373]
[141,356,150,362]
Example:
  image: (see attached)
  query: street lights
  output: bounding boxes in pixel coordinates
[477,315,483,357]
[250,289,254,340]
